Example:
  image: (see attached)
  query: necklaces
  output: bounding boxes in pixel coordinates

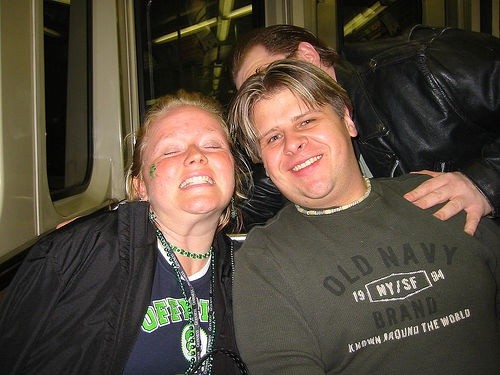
[149,215,216,375]
[295,175,371,216]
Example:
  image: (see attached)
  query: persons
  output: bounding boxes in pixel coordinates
[0,87,250,375]
[220,22,499,278]
[225,57,500,375]
